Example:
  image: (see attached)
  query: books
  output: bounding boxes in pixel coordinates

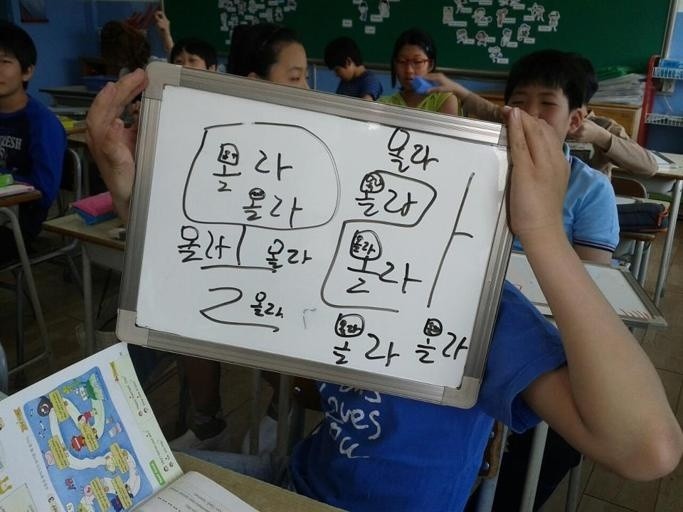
[585,72,646,106]
[0,180,36,199]
[0,338,256,512]
[643,147,680,170]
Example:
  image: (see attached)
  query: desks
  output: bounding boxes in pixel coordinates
[0,52,683,512]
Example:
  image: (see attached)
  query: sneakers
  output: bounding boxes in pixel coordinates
[167,419,230,453]
[240,403,296,455]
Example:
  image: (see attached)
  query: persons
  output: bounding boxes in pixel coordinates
[127,18,312,458]
[322,33,384,102]
[460,45,623,512]
[418,49,659,185]
[95,20,134,75]
[82,63,681,512]
[0,17,68,271]
[167,29,218,71]
[109,10,176,81]
[372,26,468,118]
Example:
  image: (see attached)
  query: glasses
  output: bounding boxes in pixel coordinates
[393,57,430,69]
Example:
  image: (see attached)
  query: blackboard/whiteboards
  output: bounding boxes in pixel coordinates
[160,0,678,96]
[115,60,515,410]
